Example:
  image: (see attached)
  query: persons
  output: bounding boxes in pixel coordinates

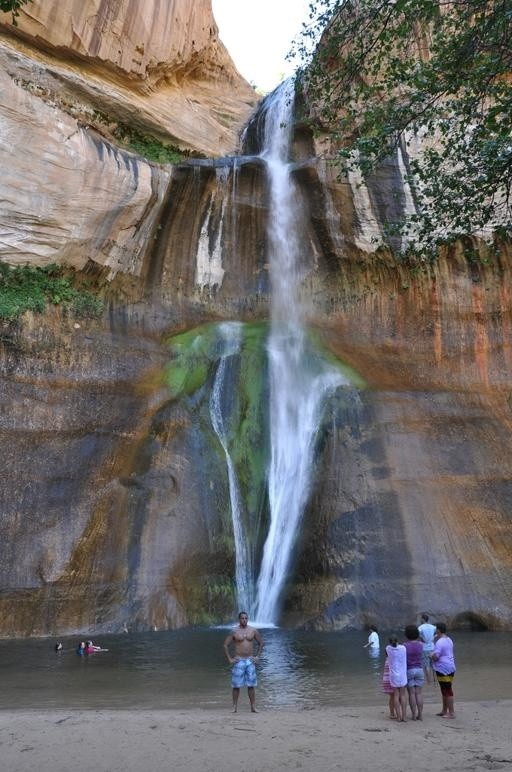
[56,643,63,650]
[77,642,86,654]
[362,614,456,722]
[223,611,265,714]
[86,640,109,654]
